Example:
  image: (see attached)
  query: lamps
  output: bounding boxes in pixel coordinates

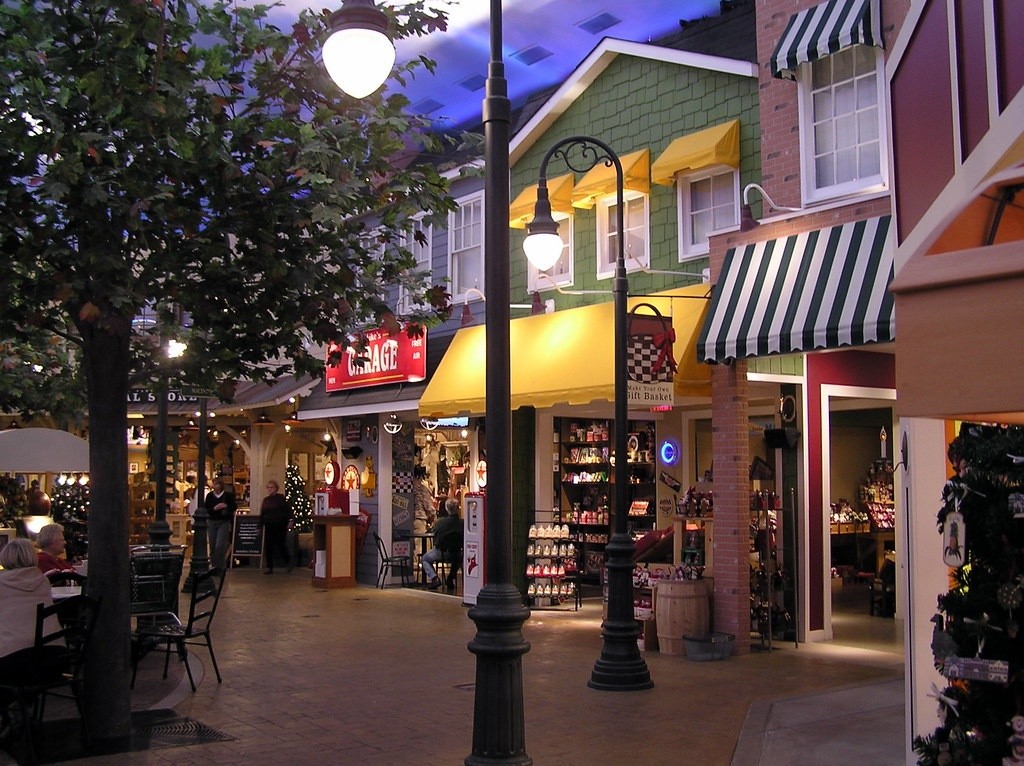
[879,426,907,475]
[739,183,802,233]
[396,249,711,325]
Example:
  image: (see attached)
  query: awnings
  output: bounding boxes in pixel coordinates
[770,0,885,83]
[298,333,455,420]
[509,172,575,230]
[651,119,742,187]
[418,284,712,417]
[0,428,91,474]
[697,213,895,366]
[0,375,322,417]
[571,148,650,210]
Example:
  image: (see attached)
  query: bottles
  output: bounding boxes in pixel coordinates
[527,542,575,557]
[526,562,565,578]
[528,582,575,596]
[529,523,569,541]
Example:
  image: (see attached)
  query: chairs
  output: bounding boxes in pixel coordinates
[373,531,411,590]
[0,542,230,766]
[435,529,465,597]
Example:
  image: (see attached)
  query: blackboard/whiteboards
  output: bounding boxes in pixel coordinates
[232,513,266,556]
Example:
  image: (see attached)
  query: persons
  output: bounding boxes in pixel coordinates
[463,449,488,468]
[3,473,39,494]
[0,538,67,720]
[35,525,77,574]
[413,462,436,571]
[257,480,296,575]
[184,475,238,576]
[422,499,464,590]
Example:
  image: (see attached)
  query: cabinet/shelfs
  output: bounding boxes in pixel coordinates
[670,518,713,584]
[748,487,799,654]
[598,581,658,652]
[525,415,683,612]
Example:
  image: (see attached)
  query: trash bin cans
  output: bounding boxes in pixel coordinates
[130,545,189,644]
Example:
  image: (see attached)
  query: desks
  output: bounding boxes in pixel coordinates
[831,500,896,579]
[412,532,436,591]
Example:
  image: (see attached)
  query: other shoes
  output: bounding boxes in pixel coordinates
[427,577,442,589]
[445,577,456,592]
[263,569,274,574]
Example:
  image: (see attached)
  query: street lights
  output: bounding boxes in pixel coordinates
[520,136,656,693]
[168,338,212,594]
[313,0,535,766]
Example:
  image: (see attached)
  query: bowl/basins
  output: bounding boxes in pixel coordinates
[682,632,736,662]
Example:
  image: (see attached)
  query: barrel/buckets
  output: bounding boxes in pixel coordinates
[655,578,709,656]
